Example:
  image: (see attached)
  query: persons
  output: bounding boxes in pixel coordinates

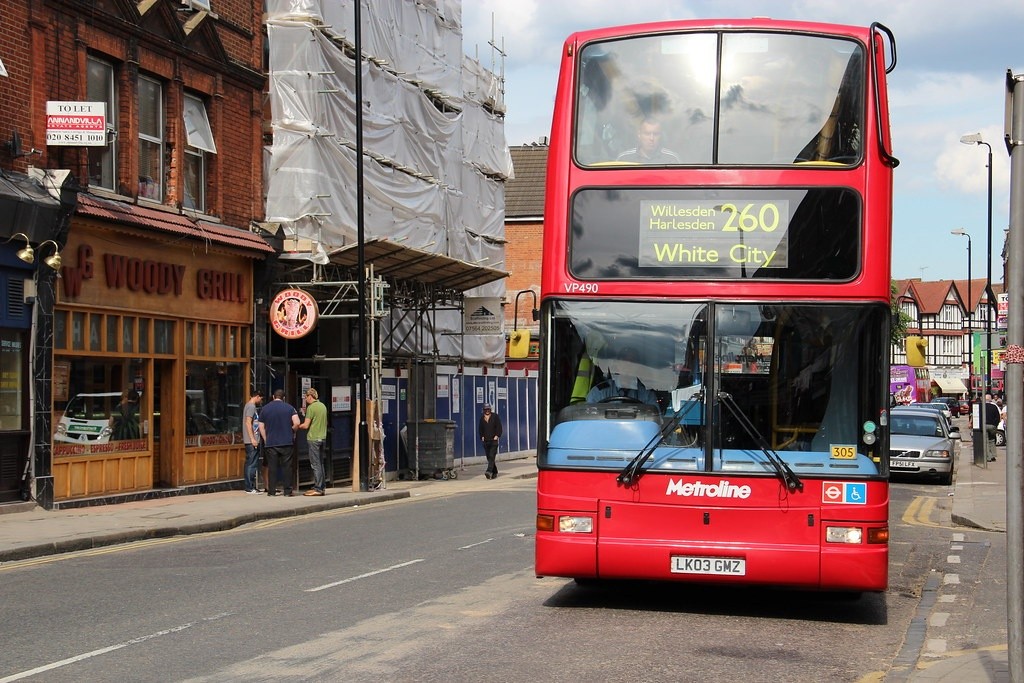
[479,403,502,479]
[615,117,680,164]
[299,388,328,496]
[587,347,665,425]
[242,390,264,494]
[258,390,300,497]
[970,394,1007,462]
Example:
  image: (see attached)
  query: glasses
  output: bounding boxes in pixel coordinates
[486,410,490,412]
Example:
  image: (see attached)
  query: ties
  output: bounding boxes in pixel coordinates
[622,388,630,403]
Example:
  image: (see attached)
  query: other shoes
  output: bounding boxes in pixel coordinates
[991,457,996,461]
[244,489,263,495]
[987,460,991,462]
[491,474,497,479]
[267,493,274,496]
[304,490,325,496]
[284,493,291,497]
[485,472,492,480]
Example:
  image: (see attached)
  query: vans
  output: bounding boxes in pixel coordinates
[52,388,207,446]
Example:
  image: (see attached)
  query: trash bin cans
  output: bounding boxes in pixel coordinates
[408,418,457,480]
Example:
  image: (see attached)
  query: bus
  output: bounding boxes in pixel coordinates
[510,18,900,600]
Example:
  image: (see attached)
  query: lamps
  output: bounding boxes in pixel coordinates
[34,240,62,270]
[0,233,35,264]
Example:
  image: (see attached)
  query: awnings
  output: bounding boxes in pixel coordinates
[933,378,968,394]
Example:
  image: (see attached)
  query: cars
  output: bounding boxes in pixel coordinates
[228,404,242,430]
[995,405,1007,447]
[958,400,970,414]
[889,403,954,485]
[930,397,960,418]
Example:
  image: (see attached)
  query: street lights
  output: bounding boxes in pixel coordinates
[949,225,974,420]
[959,129,994,392]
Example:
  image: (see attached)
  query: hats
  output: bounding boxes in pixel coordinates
[484,403,491,409]
